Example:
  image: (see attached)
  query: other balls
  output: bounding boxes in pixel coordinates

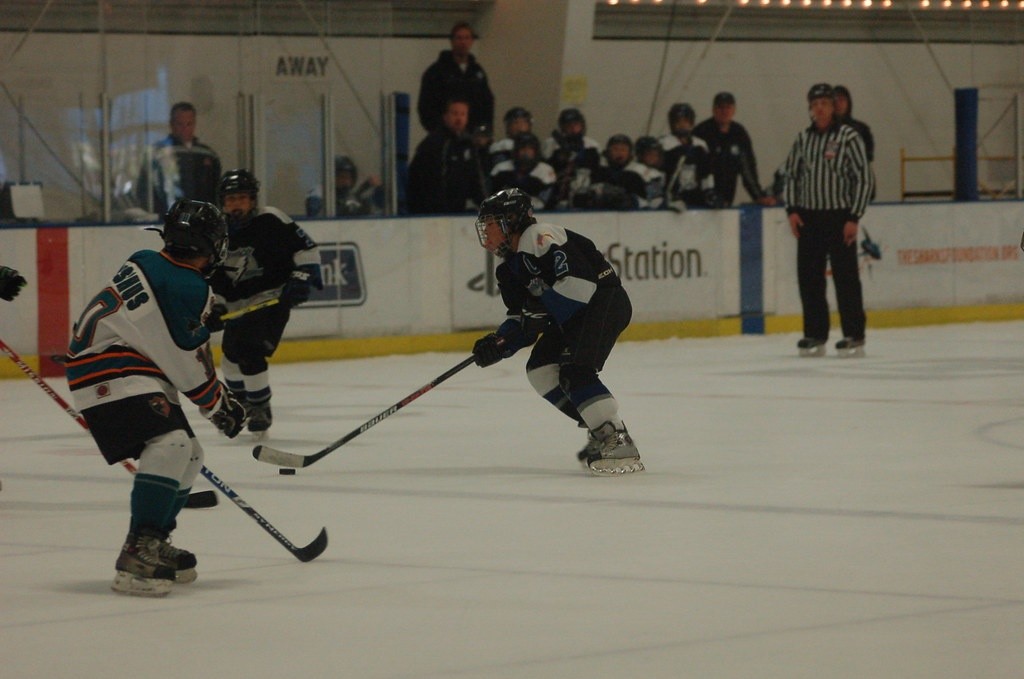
[280,469,295,475]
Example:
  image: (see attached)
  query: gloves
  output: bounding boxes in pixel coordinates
[474,332,517,367]
[204,302,231,331]
[278,277,311,310]
[520,310,556,344]
[201,382,249,440]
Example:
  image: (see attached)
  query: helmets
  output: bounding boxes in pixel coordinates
[503,108,530,125]
[513,132,538,149]
[714,93,737,104]
[333,156,356,179]
[215,170,259,222]
[604,134,631,153]
[637,135,660,152]
[471,125,492,137]
[145,196,230,269]
[667,103,696,124]
[807,84,833,102]
[474,188,537,257]
[558,110,583,128]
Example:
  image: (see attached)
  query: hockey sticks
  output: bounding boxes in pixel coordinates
[252,335,506,468]
[0,340,218,509]
[201,467,328,562]
[220,298,280,321]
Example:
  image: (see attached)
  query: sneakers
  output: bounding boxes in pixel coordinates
[112,535,175,597]
[0,267,27,301]
[225,378,274,442]
[836,336,867,359]
[154,537,199,584]
[798,336,827,357]
[578,421,645,476]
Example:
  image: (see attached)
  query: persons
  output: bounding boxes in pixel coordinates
[783,82,874,358]
[64,198,249,597]
[472,188,645,475]
[138,102,221,216]
[765,87,876,196]
[693,92,778,207]
[488,101,728,212]
[204,169,323,445]
[304,154,383,217]
[406,97,491,215]
[417,23,495,150]
